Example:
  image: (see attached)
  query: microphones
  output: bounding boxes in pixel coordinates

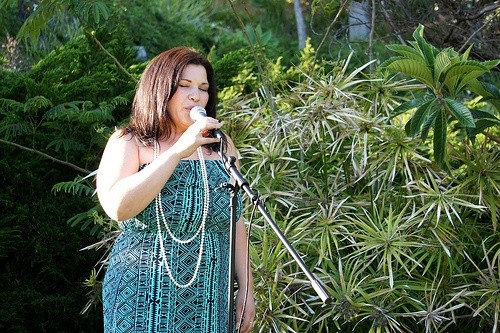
[189,106,224,140]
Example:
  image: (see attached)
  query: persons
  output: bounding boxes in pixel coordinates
[96,47,256,333]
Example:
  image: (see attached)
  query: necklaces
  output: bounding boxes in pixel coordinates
[154,137,211,289]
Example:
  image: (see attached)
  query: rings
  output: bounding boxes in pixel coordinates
[249,321,253,324]
[197,126,203,134]
